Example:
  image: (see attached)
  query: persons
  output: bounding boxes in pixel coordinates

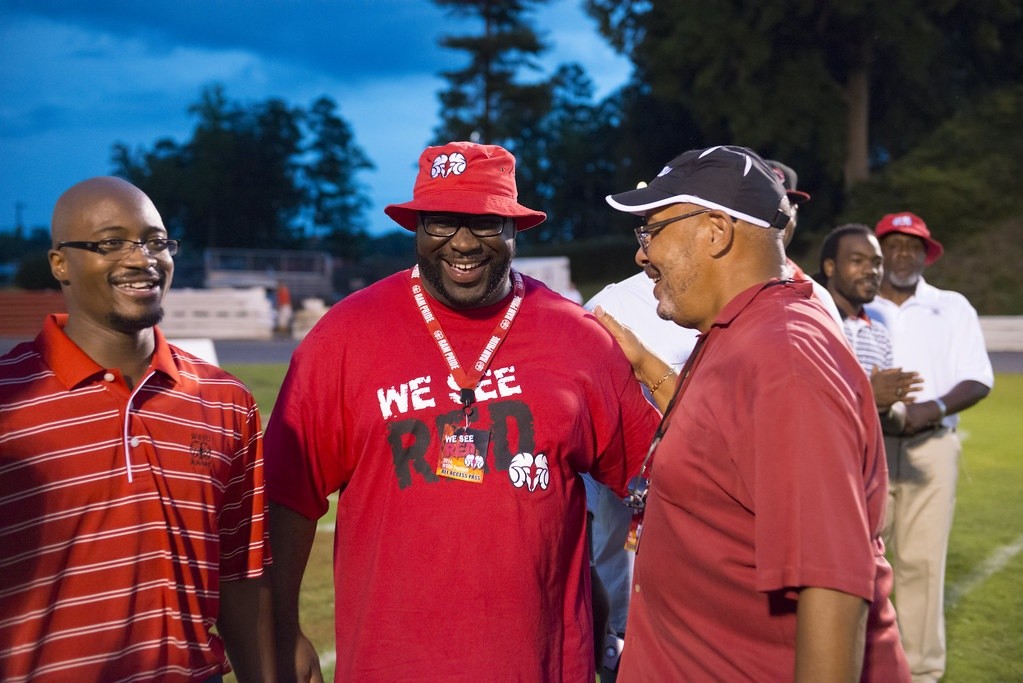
[263,143,661,683]
[579,146,922,683]
[863,211,997,682]
[0,176,273,683]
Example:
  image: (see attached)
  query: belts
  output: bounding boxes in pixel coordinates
[883,425,943,438]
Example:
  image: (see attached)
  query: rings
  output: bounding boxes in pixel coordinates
[898,387,902,395]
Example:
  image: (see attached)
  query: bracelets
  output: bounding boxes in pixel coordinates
[650,367,674,395]
[930,398,947,426]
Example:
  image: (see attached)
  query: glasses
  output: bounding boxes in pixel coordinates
[419,211,513,238]
[57,239,177,261]
[633,208,737,248]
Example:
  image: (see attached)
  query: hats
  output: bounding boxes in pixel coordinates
[606,145,790,229]
[384,141,547,232]
[764,159,811,204]
[875,211,944,267]
